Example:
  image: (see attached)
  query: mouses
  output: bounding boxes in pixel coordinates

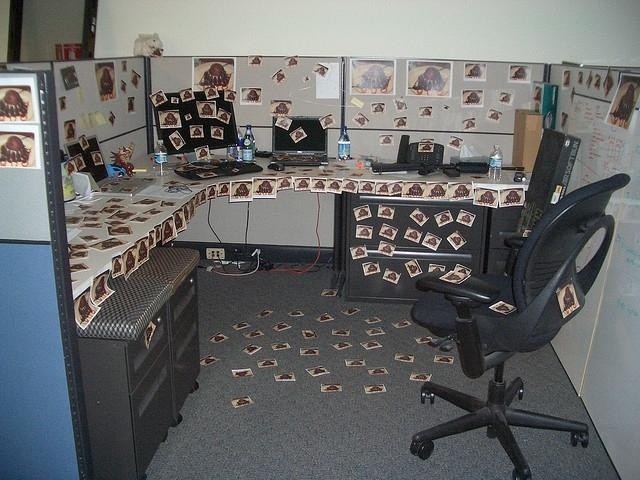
[267,163,284,171]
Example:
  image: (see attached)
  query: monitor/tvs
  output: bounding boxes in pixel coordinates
[153,90,239,158]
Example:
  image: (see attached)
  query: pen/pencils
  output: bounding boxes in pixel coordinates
[502,167,524,171]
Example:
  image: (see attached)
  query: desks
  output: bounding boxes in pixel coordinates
[64,151,526,295]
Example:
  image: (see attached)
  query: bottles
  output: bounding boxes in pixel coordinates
[488,145,502,182]
[154,139,170,176]
[338,126,351,159]
[236,125,255,161]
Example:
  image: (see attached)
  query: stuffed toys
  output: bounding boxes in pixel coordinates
[132,32,163,57]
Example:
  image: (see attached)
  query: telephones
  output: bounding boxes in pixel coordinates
[397,135,444,166]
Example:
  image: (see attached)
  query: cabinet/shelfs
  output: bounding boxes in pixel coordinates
[489,193,523,279]
[76,263,201,480]
[344,193,487,302]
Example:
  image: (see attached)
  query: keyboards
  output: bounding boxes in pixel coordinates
[174,160,263,181]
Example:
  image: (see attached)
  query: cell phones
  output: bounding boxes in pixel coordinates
[513,172,524,182]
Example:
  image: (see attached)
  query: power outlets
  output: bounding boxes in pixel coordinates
[206,248,227,262]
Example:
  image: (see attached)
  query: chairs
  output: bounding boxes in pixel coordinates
[407,175,633,479]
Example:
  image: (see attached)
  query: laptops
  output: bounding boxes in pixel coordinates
[64,134,155,197]
[272,116,328,166]
